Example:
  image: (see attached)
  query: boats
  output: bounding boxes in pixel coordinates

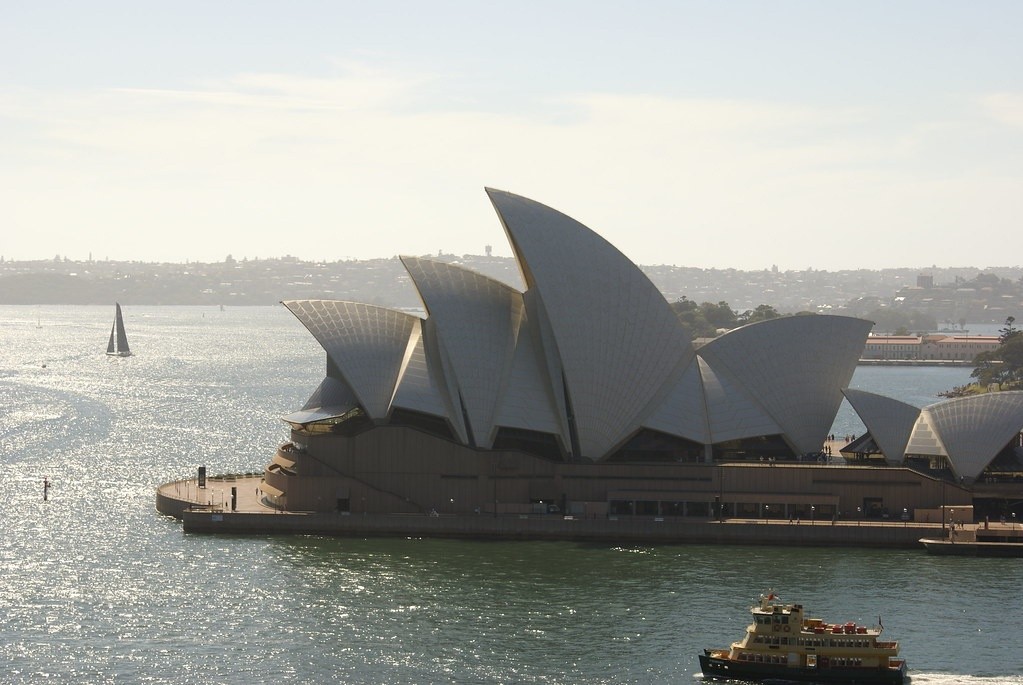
[698,589,908,685]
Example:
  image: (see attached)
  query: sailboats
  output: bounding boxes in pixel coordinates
[106,302,131,357]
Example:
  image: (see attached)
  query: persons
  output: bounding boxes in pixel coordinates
[760,456,764,465]
[955,520,962,529]
[769,457,777,466]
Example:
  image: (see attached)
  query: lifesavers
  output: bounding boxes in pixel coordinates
[774,624,781,632]
[783,625,791,633]
